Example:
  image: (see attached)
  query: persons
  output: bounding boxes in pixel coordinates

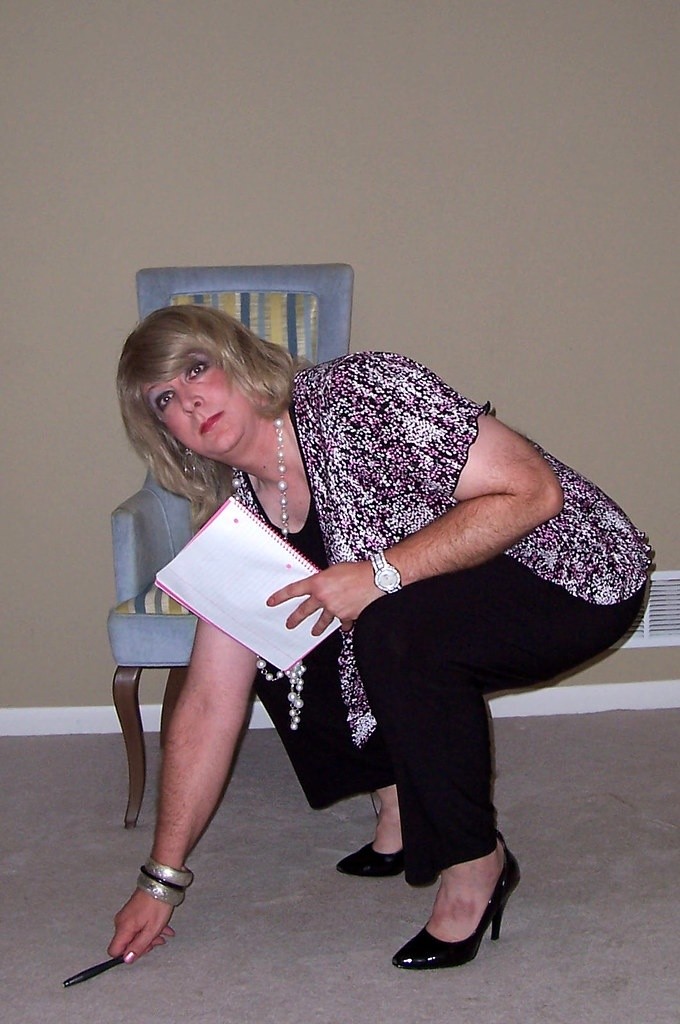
[106,307,652,968]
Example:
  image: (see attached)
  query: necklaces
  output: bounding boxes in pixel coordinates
[231,414,308,731]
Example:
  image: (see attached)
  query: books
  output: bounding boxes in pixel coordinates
[153,496,342,671]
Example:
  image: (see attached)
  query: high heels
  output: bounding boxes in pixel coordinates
[336,842,404,877]
[392,830,520,970]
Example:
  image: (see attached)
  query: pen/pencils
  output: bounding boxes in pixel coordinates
[61,954,126,989]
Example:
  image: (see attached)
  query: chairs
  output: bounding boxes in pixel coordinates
[107,263,354,827]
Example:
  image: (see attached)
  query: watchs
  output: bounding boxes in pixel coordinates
[369,550,402,594]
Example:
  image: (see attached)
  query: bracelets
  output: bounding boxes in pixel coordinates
[136,858,194,907]
